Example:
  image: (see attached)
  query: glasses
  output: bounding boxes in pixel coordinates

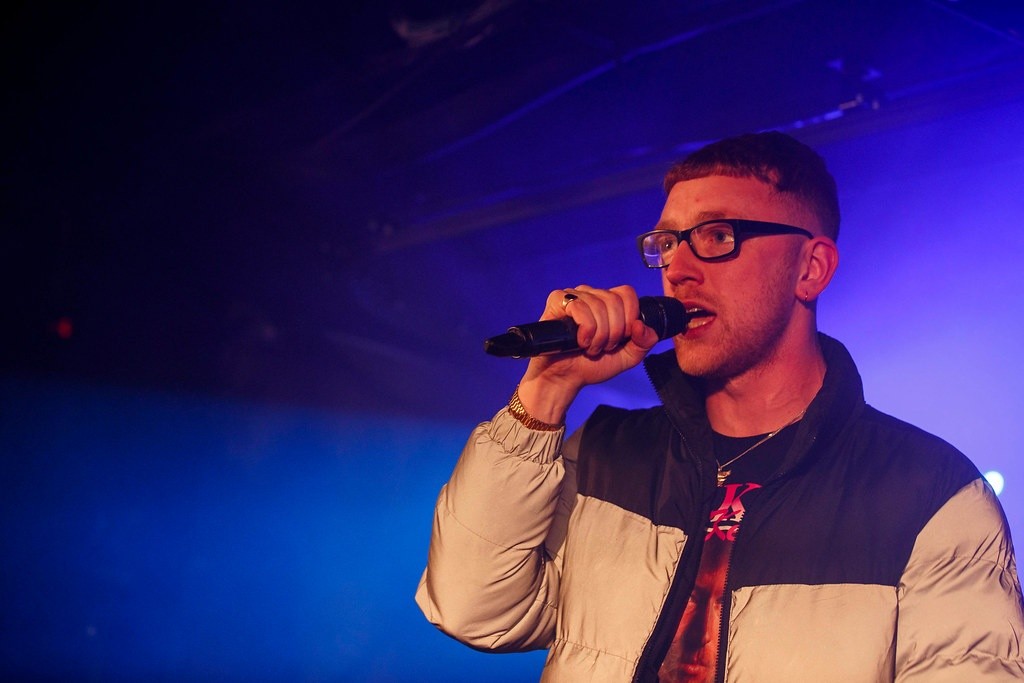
[636,220,813,270]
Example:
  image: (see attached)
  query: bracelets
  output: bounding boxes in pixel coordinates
[508,384,563,430]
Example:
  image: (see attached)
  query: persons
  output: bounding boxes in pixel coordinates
[413,131,1023,683]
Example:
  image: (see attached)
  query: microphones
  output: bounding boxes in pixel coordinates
[483,296,688,360]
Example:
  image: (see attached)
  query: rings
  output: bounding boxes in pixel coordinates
[562,294,578,311]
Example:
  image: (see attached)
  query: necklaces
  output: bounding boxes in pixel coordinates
[714,403,807,488]
